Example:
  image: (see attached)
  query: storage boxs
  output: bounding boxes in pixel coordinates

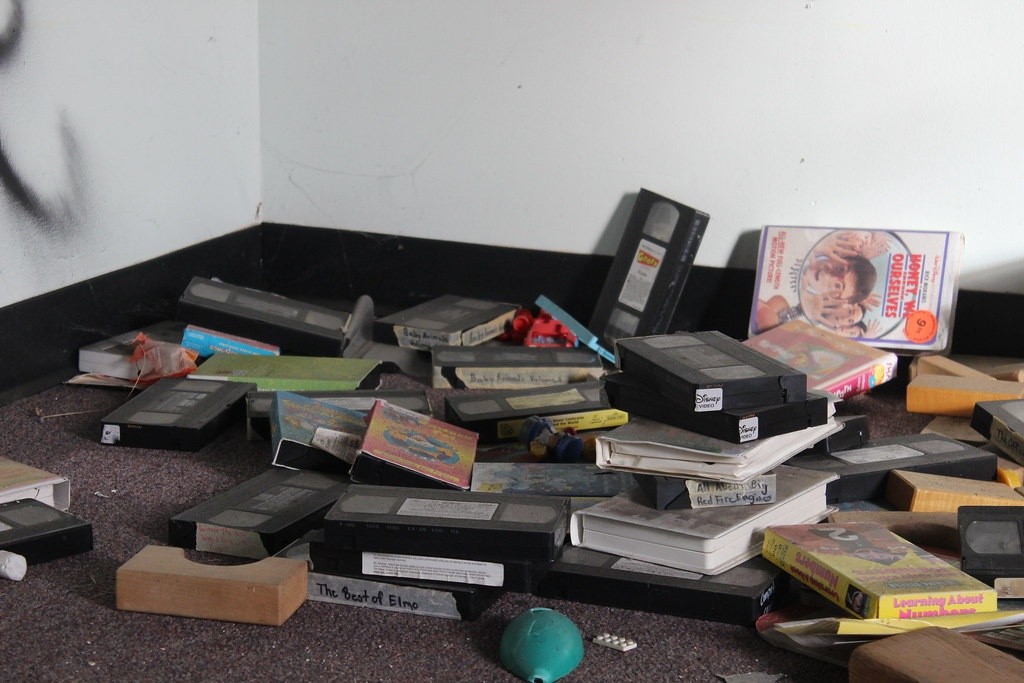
[0,189,1024,683]
